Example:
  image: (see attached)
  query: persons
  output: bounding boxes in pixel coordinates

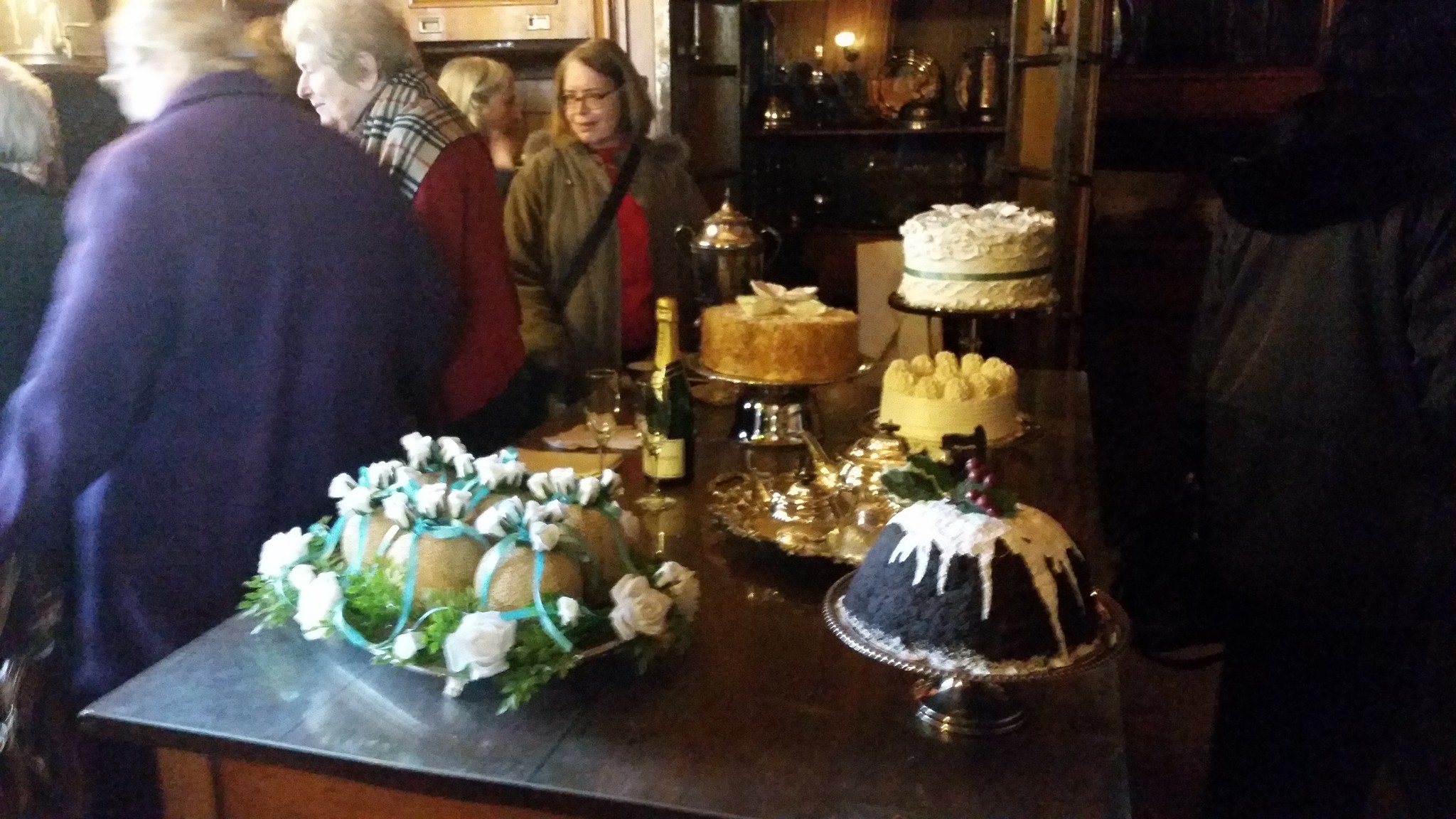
[438,54,518,202]
[1194,17,1454,819]
[0,56,77,396]
[279,0,529,422]
[502,39,712,420]
[0,0,465,818]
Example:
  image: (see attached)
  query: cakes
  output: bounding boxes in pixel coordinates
[841,488,1108,662]
[872,347,1021,447]
[889,194,1056,306]
[692,277,862,380]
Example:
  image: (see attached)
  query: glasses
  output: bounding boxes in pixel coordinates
[559,84,620,112]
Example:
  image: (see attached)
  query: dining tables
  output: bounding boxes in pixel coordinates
[72,349,1127,819]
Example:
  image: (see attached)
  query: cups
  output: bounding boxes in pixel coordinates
[640,296,696,490]
[675,185,787,311]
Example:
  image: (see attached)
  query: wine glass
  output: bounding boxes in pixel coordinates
[582,369,621,484]
[633,374,675,510]
